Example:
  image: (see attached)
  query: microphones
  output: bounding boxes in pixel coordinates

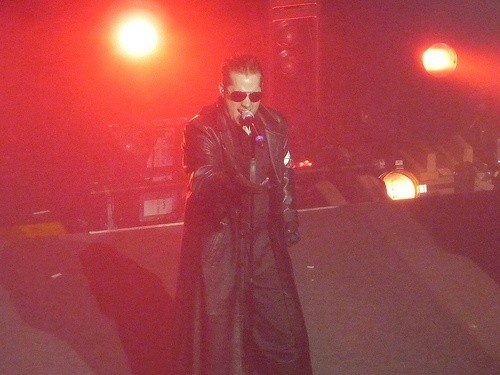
[241,110,264,147]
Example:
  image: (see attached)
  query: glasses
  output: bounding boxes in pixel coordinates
[224,86,262,103]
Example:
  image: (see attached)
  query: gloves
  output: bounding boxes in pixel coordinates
[284,225,303,247]
[235,173,272,194]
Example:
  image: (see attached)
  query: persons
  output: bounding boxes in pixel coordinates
[308,145,385,208]
[175,56,312,375]
